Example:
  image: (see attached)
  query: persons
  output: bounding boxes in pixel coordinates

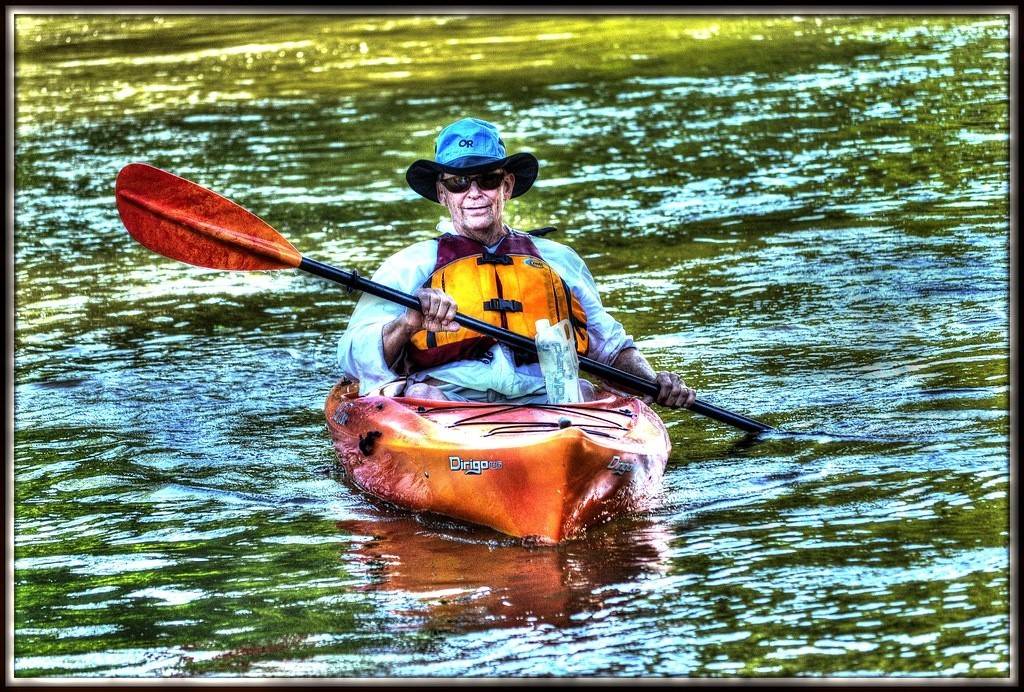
[336,117,696,412]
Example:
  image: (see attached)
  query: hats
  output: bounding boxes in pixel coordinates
[406,118,538,204]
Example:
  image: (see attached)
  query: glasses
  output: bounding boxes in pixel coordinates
[436,171,505,193]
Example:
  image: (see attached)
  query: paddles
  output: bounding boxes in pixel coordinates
[115,162,774,432]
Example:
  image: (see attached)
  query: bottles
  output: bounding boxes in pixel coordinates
[535,319,571,404]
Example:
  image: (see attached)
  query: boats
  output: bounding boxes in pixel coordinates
[324,377,674,548]
